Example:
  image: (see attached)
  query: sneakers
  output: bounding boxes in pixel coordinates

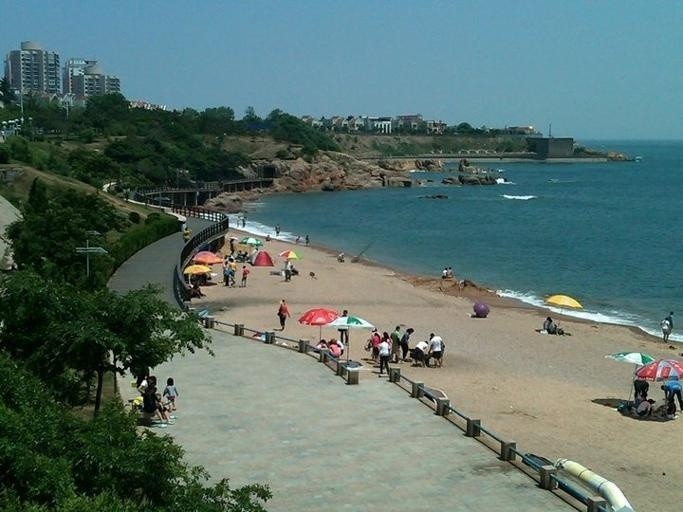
[158,409,177,428]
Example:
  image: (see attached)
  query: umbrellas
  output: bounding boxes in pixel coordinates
[603,350,654,401]
[633,357,683,407]
[297,308,338,342]
[543,294,583,326]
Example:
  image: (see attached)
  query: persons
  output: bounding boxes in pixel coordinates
[660,381,683,412]
[276,300,291,330]
[657,398,678,420]
[315,309,445,367]
[662,311,674,340]
[142,384,174,424]
[181,215,346,298]
[660,317,671,343]
[137,376,156,394]
[161,377,178,412]
[632,379,650,403]
[636,398,654,417]
[447,267,453,278]
[542,315,570,337]
[441,268,447,279]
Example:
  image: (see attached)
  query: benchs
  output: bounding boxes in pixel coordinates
[422,386,452,415]
[132,405,154,425]
